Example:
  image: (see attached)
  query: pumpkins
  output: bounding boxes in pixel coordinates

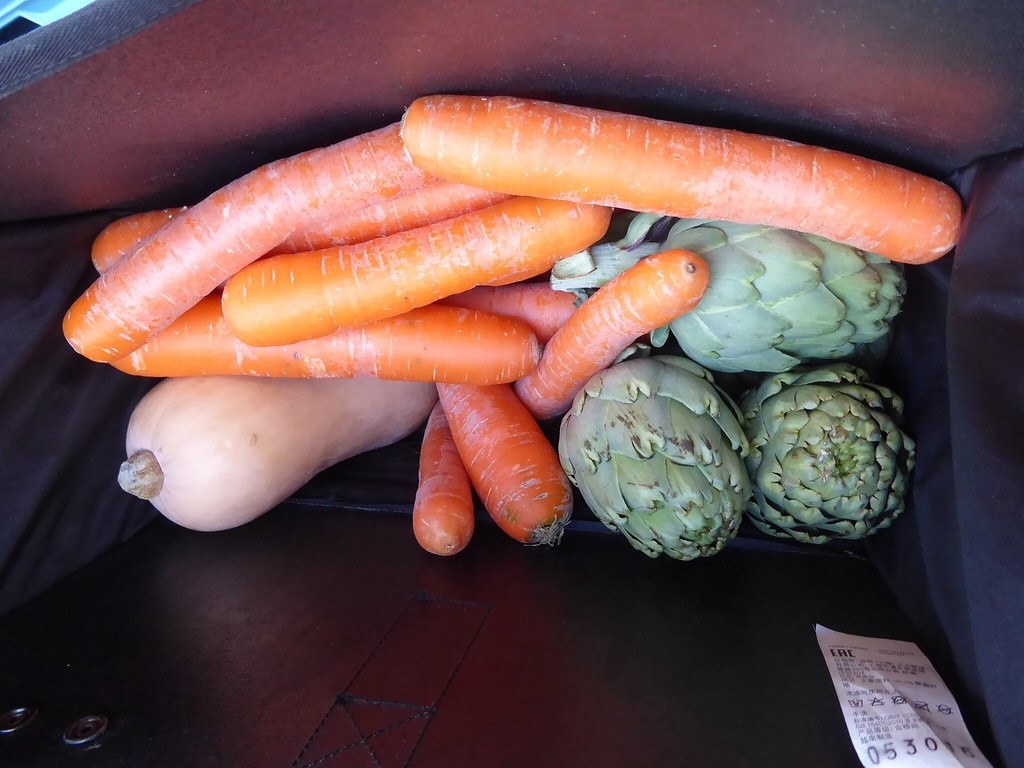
[117,377,434,529]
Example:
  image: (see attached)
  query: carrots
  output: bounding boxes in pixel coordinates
[63,95,962,557]
